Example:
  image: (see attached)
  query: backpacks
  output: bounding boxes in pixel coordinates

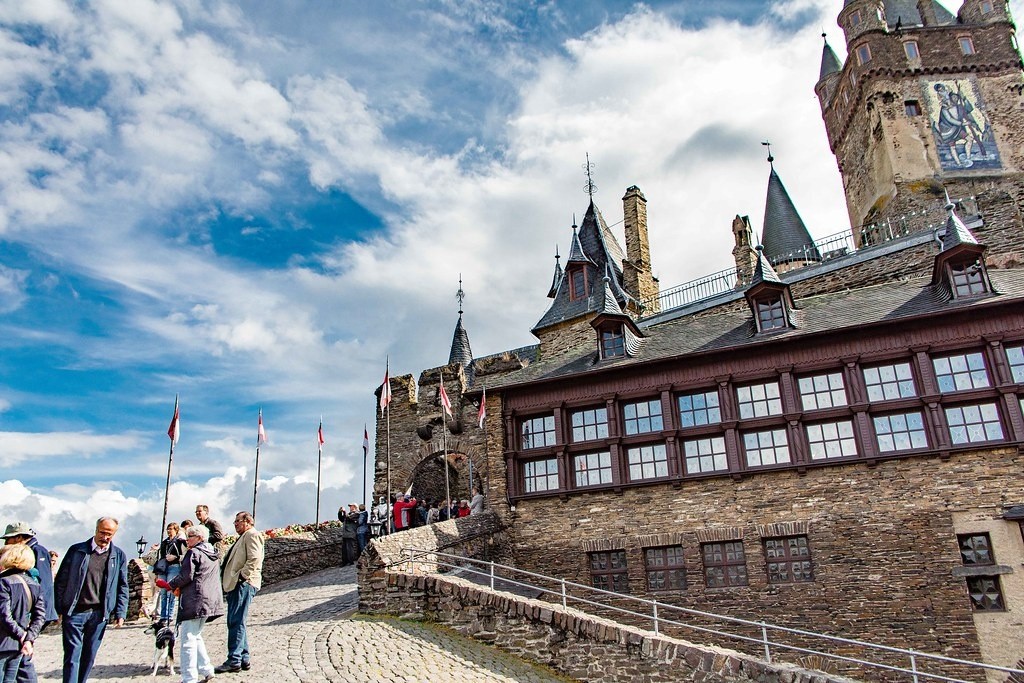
[430,508,439,524]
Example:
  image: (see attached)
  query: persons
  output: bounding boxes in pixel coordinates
[178,520,193,541]
[358,504,368,552]
[54,516,130,683]
[196,504,223,566]
[213,511,264,673]
[0,521,58,683]
[338,503,360,567]
[167,525,224,683]
[159,522,187,624]
[376,485,484,535]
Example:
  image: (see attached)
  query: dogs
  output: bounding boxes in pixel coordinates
[143,622,175,676]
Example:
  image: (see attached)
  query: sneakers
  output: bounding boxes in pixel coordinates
[241,662,250,670]
[214,661,241,673]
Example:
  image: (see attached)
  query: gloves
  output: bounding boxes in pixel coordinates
[156,579,171,591]
[173,588,179,595]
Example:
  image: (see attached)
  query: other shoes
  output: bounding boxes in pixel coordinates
[205,672,214,681]
[349,562,354,565]
[339,563,348,567]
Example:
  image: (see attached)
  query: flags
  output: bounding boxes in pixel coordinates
[363,430,369,454]
[404,484,413,497]
[318,426,324,448]
[380,369,391,417]
[258,410,268,446]
[478,389,486,427]
[440,382,453,421]
[168,398,180,445]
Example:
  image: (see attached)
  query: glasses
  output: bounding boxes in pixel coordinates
[233,519,247,523]
[97,529,113,538]
[195,510,204,513]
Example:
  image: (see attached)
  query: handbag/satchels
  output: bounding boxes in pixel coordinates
[152,557,167,574]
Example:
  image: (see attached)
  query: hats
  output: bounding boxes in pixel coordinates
[396,492,404,499]
[0,522,35,539]
[348,503,358,509]
